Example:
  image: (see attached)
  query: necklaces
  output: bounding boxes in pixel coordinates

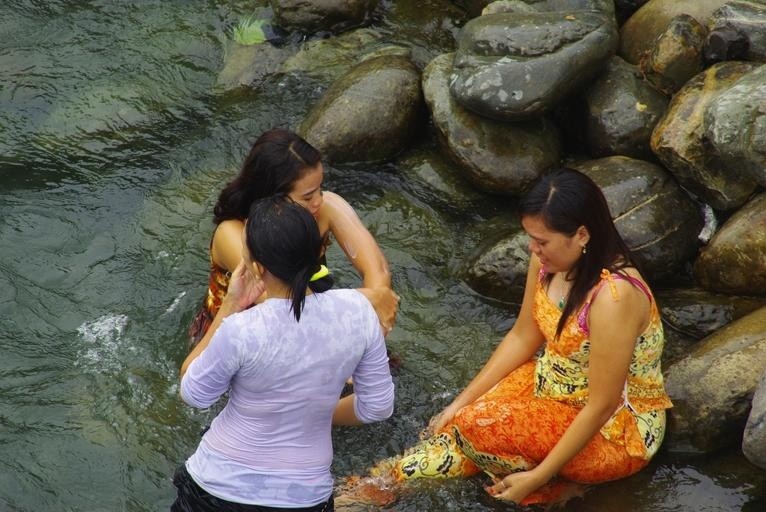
[560,271,567,308]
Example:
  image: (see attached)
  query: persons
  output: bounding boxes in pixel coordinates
[332,167,674,512]
[187,128,400,396]
[171,193,395,511]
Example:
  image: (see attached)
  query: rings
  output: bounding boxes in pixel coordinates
[500,482,505,487]
